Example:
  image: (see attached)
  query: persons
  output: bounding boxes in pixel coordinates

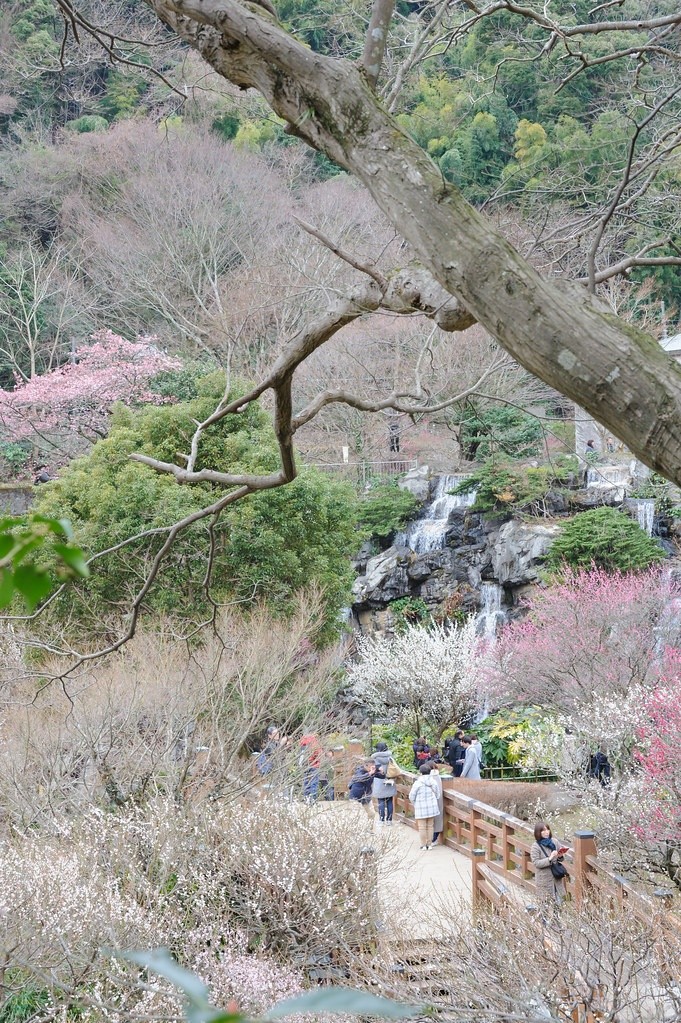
[412,730,485,780]
[32,464,51,486]
[408,764,441,852]
[425,760,444,847]
[586,439,596,453]
[605,437,615,452]
[246,727,343,802]
[529,822,568,929]
[370,742,399,826]
[348,758,387,836]
[585,742,612,793]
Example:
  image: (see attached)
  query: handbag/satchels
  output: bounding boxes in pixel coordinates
[551,862,567,880]
[480,761,487,769]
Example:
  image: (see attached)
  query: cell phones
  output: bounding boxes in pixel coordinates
[558,847,569,854]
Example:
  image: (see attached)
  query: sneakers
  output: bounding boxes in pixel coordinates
[417,845,427,850]
[425,846,432,850]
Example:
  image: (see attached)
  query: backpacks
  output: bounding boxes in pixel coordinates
[386,756,402,778]
[298,739,316,770]
[257,739,273,774]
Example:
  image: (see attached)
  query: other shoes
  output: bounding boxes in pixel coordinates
[430,840,438,846]
